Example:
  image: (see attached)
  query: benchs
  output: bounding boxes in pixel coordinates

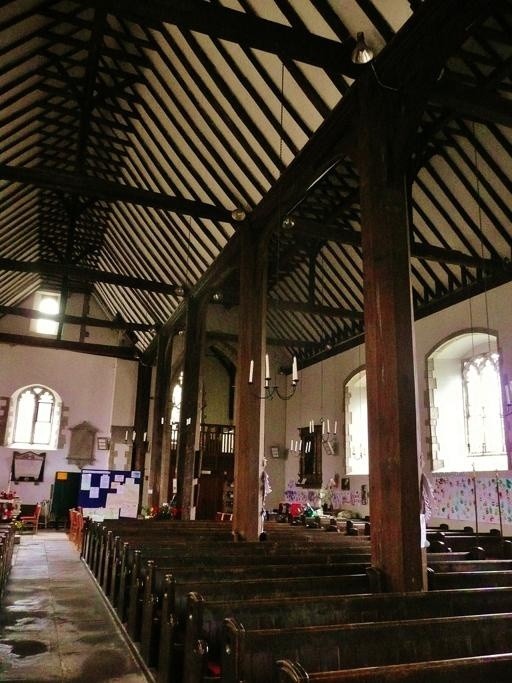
[79,512,510,682]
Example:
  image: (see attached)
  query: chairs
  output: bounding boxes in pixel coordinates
[20,503,42,534]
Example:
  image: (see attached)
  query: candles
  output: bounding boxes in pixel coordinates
[242,351,299,390]
[290,416,337,454]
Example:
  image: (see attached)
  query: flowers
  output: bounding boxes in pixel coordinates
[143,499,178,520]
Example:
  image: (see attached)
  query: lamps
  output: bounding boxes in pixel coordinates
[349,29,401,90]
[230,202,256,230]
